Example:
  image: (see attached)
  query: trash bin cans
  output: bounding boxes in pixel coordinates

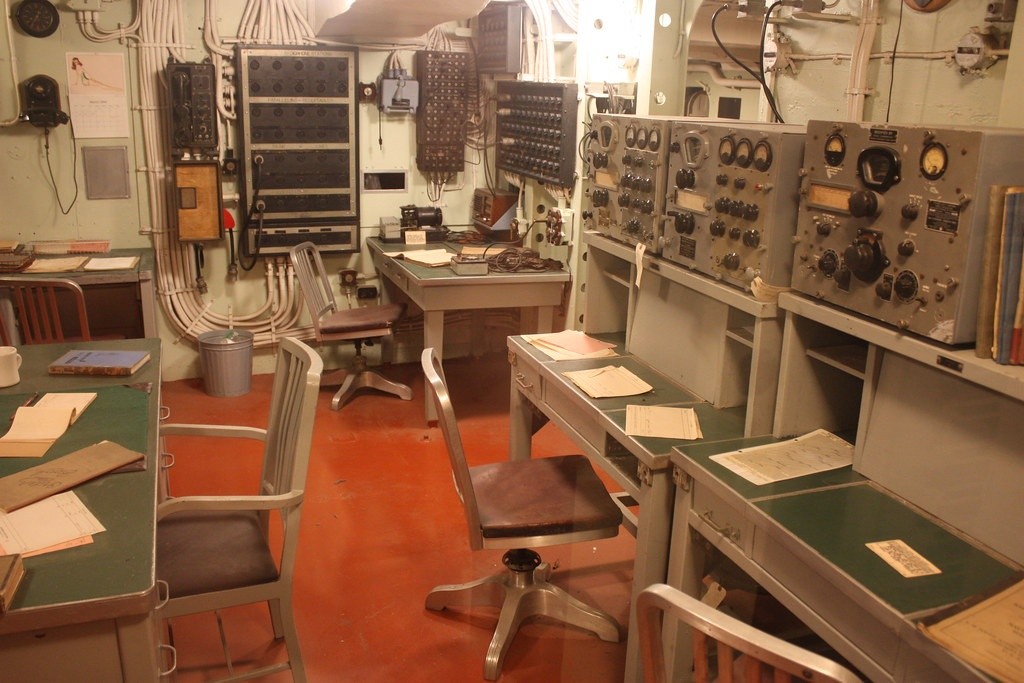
[195,329,255,398]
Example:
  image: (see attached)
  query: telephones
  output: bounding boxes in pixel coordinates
[166,63,219,148]
[17,74,69,128]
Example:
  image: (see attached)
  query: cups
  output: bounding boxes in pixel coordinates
[0,347,23,388]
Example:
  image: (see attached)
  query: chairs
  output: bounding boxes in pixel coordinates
[156,336,325,683]
[636,583,863,683]
[0,276,128,350]
[420,346,624,683]
[291,242,414,411]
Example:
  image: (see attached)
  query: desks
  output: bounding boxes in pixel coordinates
[0,339,164,683]
[667,431,1024,683]
[506,331,746,683]
[0,247,160,339]
[368,235,572,426]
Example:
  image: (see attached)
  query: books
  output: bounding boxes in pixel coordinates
[0,240,140,274]
[916,573,1024,683]
[383,248,459,268]
[47,349,152,376]
[0,392,147,619]
[974,182,1024,366]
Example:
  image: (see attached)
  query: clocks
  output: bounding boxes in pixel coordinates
[16,0,60,38]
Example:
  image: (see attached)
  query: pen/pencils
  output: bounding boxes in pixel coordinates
[10,392,39,420]
